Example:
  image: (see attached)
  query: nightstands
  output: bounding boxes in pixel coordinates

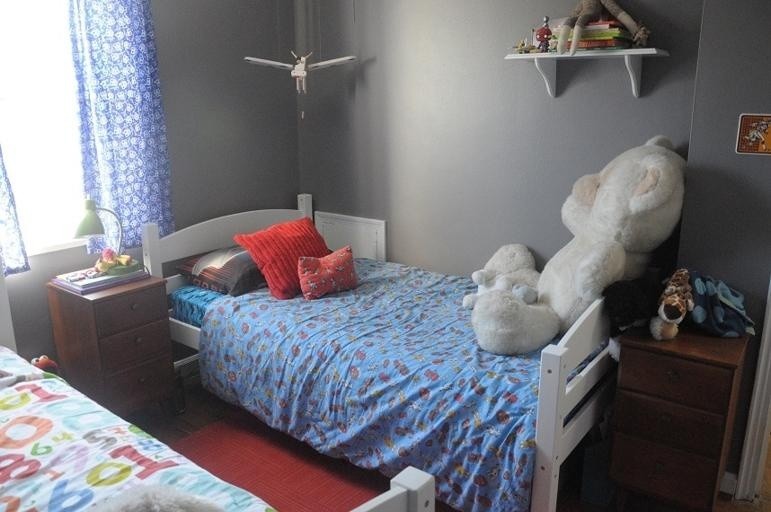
[46,273,175,418]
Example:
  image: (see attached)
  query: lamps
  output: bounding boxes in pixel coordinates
[73,200,139,274]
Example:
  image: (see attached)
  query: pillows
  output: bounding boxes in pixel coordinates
[234,217,331,299]
[175,247,267,296]
[298,244,356,300]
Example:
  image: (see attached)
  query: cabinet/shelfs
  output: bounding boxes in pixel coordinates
[605,324,750,512]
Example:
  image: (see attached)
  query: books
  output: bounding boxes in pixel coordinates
[548,19,632,50]
[50,263,151,295]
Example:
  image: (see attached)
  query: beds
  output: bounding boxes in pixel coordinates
[0,253,436,512]
[139,193,618,512]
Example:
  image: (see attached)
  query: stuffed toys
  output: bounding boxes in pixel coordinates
[31,355,58,372]
[512,16,552,54]
[462,135,695,354]
[556,0,650,55]
[462,135,694,356]
[94,247,132,272]
